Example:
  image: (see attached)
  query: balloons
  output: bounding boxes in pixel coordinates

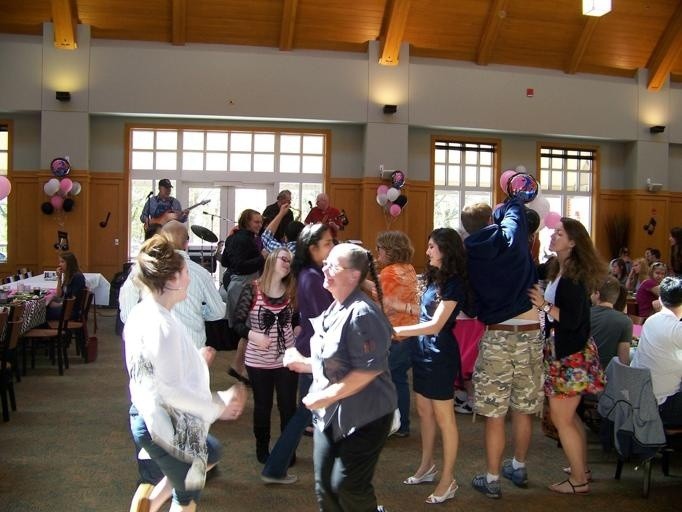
[376,170,408,217]
[493,166,560,235]
[0,175,12,199]
[41,155,81,214]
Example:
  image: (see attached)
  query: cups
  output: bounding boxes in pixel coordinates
[45,271,49,278]
[3,286,10,292]
[18,281,24,293]
[34,287,40,294]
[23,284,31,293]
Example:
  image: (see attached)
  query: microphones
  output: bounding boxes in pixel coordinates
[202,211,211,215]
[308,201,313,208]
[145,191,153,199]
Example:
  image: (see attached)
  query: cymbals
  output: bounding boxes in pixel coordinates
[191,225,218,242]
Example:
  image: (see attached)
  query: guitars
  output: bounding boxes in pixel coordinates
[169,200,210,223]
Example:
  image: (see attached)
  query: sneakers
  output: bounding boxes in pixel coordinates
[227,368,250,388]
[260,474,297,484]
[256,450,271,464]
[472,475,502,499]
[288,453,296,468]
[501,461,528,488]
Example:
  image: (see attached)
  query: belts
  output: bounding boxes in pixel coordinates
[484,323,540,332]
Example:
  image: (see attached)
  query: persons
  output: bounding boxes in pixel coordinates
[119,220,244,512]
[140,179,189,240]
[46,251,86,356]
[530,216,682,493]
[457,202,541,496]
[223,190,398,512]
[361,228,466,503]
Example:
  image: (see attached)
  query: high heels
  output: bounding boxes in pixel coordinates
[426,480,458,504]
[404,465,438,485]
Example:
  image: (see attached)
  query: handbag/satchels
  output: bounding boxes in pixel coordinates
[85,337,97,362]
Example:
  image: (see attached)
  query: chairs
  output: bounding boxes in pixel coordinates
[598,290,682,494]
[0,271,95,424]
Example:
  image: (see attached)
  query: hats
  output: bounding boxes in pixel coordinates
[159,179,173,188]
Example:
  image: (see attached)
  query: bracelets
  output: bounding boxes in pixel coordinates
[55,275,61,278]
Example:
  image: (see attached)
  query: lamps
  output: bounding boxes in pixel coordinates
[581,0,612,17]
[650,126,665,134]
[56,92,70,102]
[384,104,397,114]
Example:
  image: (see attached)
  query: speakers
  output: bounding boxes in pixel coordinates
[379,164,395,180]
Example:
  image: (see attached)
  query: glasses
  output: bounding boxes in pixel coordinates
[277,256,291,264]
[377,246,381,252]
[653,263,667,267]
[322,261,352,272]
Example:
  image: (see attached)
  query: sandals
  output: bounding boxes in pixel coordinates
[564,466,591,481]
[548,478,590,495]
